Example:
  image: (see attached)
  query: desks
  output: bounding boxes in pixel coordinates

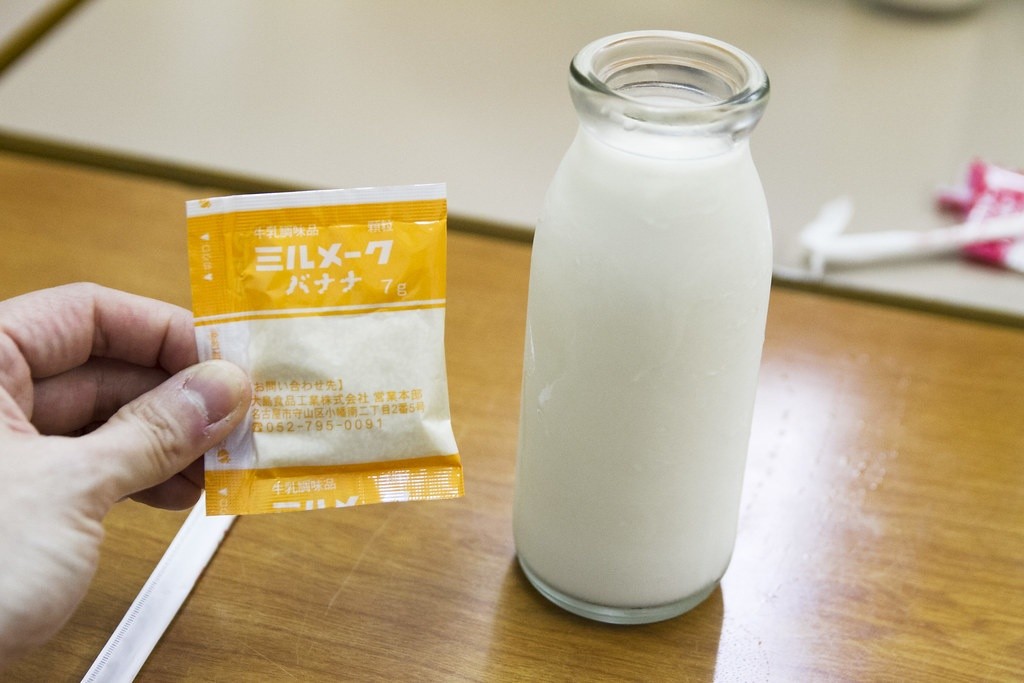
[0,130,1024,682]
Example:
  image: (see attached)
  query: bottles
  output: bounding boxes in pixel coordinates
[514,29,774,627]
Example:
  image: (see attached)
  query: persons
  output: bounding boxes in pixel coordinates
[0,281,253,667]
